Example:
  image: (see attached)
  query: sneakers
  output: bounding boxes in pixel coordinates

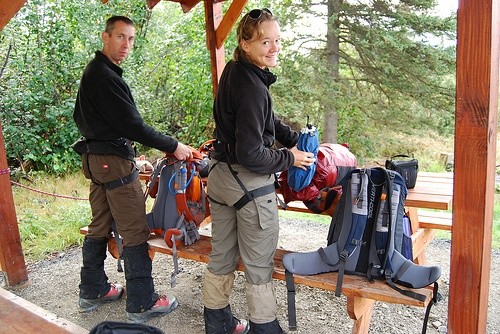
[126,293,178,324]
[231,317,250,334]
[79,283,124,313]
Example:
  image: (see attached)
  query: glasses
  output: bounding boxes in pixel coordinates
[243,6,274,28]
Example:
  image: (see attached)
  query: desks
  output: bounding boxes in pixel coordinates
[139,172,454,334]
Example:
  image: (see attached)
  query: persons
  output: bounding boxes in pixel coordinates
[71,16,193,324]
[204,8,316,334]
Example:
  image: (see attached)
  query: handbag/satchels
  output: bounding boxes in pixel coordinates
[384,154,418,191]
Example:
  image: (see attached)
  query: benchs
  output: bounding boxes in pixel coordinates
[80,225,443,307]
[277,199,453,230]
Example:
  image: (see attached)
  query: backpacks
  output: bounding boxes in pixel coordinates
[282,166,442,288]
[275,142,357,214]
[145,151,210,248]
